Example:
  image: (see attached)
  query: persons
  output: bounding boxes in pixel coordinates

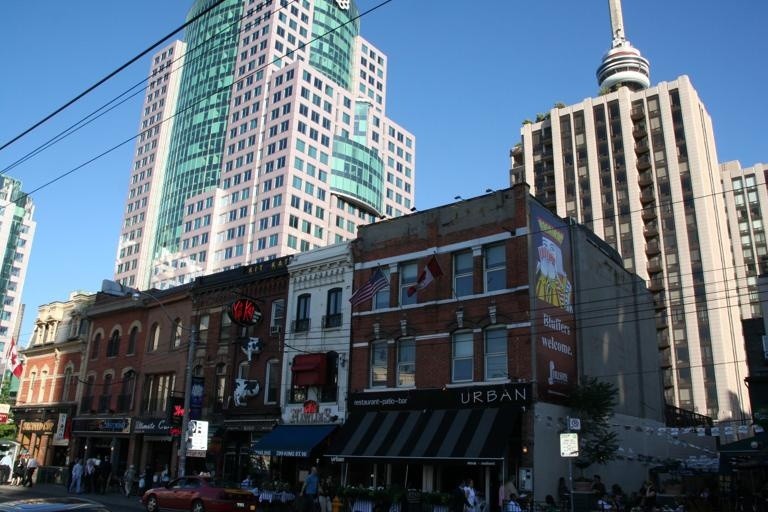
[242,474,253,483]
[22,455,38,487]
[318,475,333,512]
[590,475,606,494]
[557,475,571,512]
[640,479,657,512]
[458,478,479,512]
[503,474,521,501]
[0,451,13,485]
[609,483,627,506]
[10,455,24,486]
[505,494,523,512]
[67,453,170,498]
[298,465,324,512]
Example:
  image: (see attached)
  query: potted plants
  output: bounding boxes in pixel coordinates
[343,486,452,512]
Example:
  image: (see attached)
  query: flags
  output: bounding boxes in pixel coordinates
[670,426,681,437]
[645,426,655,437]
[695,427,709,438]
[724,426,736,436]
[250,332,283,371]
[656,427,669,437]
[348,266,390,308]
[4,335,24,379]
[738,425,749,434]
[682,427,693,436]
[753,423,765,433]
[598,421,645,433]
[407,254,444,297]
[710,426,722,436]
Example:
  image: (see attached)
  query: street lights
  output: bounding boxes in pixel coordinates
[131,288,198,476]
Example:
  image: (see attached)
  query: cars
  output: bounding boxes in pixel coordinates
[141,475,261,510]
[0,496,113,512]
